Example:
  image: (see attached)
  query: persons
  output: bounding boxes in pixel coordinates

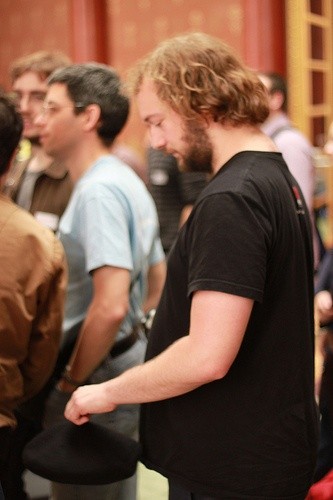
[252,67,320,274]
[0,97,69,500]
[35,62,166,500]
[64,33,333,500]
[148,124,207,254]
[8,51,73,237]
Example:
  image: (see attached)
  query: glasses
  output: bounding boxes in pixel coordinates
[3,91,44,107]
[41,104,86,114]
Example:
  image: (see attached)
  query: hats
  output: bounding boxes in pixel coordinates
[24,420,142,486]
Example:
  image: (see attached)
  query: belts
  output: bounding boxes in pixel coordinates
[106,325,140,360]
